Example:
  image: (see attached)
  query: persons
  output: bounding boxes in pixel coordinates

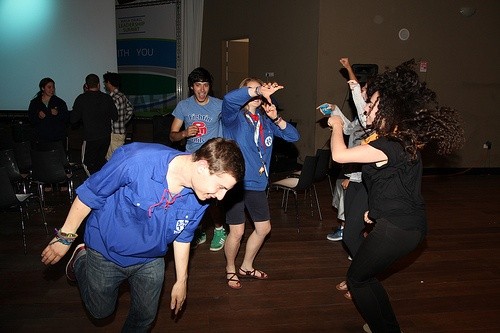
[42,137,244,333]
[315,57,458,333]
[28,72,134,178]
[222,77,300,289]
[170,67,228,251]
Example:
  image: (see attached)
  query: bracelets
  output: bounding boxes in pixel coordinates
[49,228,78,245]
[256,87,260,96]
[274,117,282,125]
[273,116,279,122]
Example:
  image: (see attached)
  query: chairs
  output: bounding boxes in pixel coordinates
[267,148,336,227]
[0,145,76,254]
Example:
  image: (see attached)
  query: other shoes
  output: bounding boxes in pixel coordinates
[336,280,352,299]
[65,244,86,281]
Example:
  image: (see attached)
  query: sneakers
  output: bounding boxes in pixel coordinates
[326,228,343,241]
[191,232,207,249]
[209,228,228,252]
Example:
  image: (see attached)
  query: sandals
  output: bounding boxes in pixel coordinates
[225,268,243,290]
[238,266,269,280]
